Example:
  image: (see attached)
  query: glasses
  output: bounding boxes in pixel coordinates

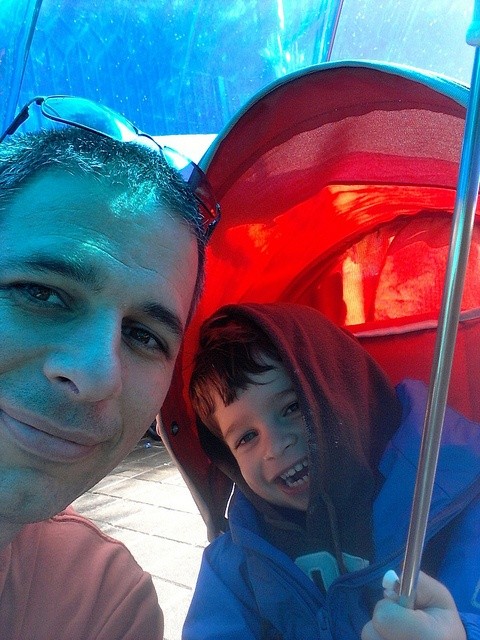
[0,95,222,244]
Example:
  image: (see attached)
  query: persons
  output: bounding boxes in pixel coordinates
[1,113,207,640]
[181,300,480,640]
